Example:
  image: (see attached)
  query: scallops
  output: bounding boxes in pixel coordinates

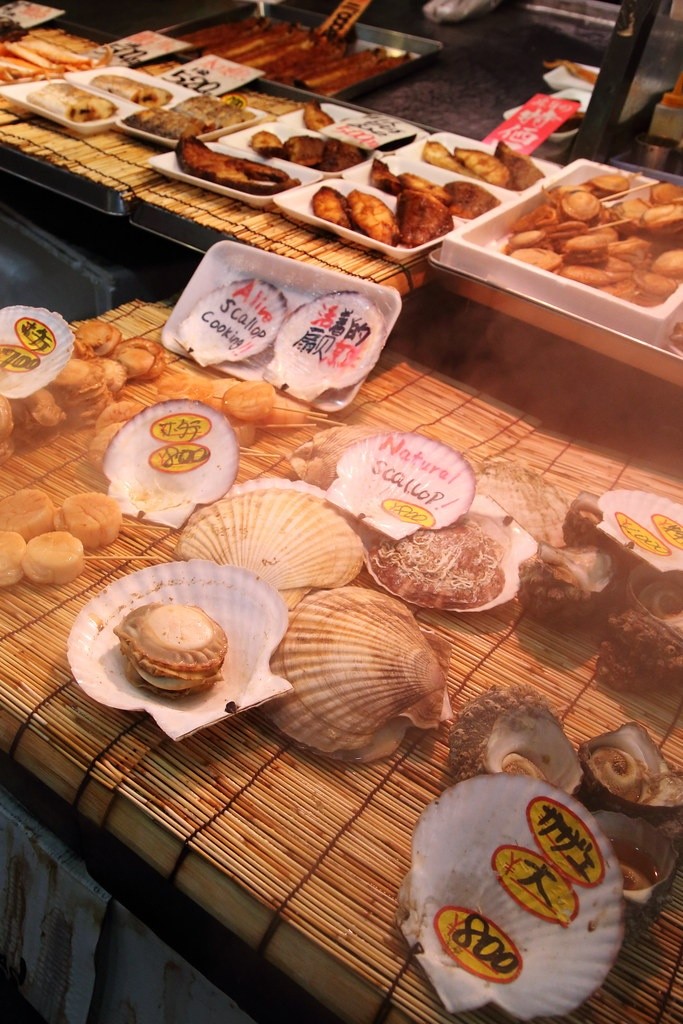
[1,279,683,1021]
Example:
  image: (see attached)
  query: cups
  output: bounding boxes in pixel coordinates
[633,134,678,173]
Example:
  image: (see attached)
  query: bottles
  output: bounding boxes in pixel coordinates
[647,71,683,147]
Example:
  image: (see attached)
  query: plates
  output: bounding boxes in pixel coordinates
[503,88,592,143]
[271,129,561,258]
[543,63,601,92]
[146,122,383,207]
[0,66,266,150]
